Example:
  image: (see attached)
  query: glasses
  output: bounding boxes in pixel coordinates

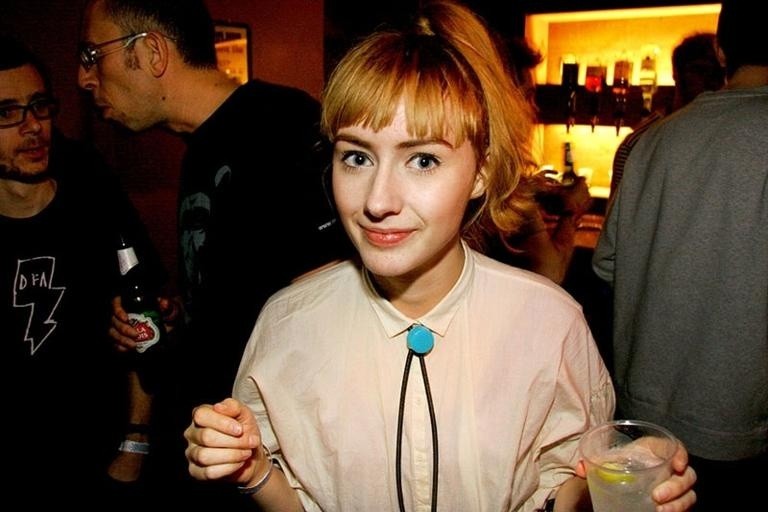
[0,95,58,129]
[78,29,178,72]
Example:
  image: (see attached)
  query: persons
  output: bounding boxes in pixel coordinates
[467,35,593,286]
[589,0,768,512]
[73,0,355,512]
[607,30,726,200]
[0,38,168,511]
[183,0,701,512]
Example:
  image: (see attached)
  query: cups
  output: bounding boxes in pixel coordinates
[580,418,678,512]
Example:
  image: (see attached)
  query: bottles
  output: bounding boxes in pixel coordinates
[582,58,606,115]
[560,140,580,187]
[612,54,631,116]
[638,51,658,112]
[113,228,177,394]
[561,58,580,116]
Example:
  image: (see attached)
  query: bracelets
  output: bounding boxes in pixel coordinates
[122,423,153,435]
[234,442,274,495]
[118,439,151,455]
